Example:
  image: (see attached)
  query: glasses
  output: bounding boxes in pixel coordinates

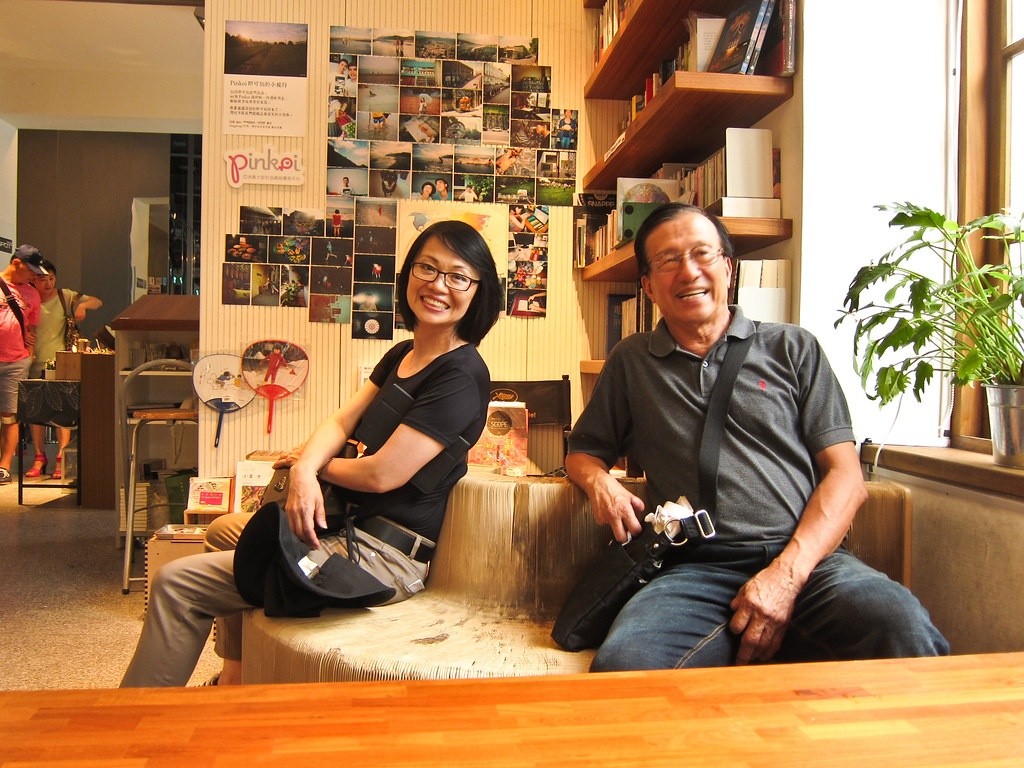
[411,261,480,291]
[647,244,727,272]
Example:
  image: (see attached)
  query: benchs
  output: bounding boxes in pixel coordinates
[237,470,918,684]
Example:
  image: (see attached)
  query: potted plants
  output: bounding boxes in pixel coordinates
[831,196,1022,469]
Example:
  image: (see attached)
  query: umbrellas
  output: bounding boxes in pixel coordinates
[419,93,432,103]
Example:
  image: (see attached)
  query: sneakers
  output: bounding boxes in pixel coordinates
[0,468,12,485]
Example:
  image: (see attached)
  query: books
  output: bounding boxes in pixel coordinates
[234,460,275,514]
[573,0,797,354]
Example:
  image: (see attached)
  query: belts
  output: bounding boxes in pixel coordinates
[352,515,433,564]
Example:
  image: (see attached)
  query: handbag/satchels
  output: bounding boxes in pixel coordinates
[57,288,83,352]
[550,517,672,653]
[259,442,358,538]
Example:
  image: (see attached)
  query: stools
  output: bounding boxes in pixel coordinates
[122,407,198,593]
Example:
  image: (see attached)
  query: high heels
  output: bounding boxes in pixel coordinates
[25,451,48,477]
[51,453,68,479]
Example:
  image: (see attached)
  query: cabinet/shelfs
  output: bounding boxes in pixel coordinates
[572,0,812,376]
[109,291,199,550]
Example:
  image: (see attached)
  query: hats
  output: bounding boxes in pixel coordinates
[14,244,49,275]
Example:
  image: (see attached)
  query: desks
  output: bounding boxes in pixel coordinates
[0,648,1024,768]
[17,378,81,504]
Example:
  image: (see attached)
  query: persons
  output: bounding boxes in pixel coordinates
[536,121,550,148]
[325,241,337,261]
[395,39,404,51]
[412,178,452,200]
[509,205,529,232]
[260,343,288,384]
[330,59,357,97]
[566,202,949,673]
[0,244,49,484]
[419,98,426,114]
[559,110,577,148]
[331,210,342,236]
[29,260,103,480]
[338,177,353,196]
[328,96,344,137]
[459,185,478,202]
[119,219,505,689]
[528,292,547,313]
